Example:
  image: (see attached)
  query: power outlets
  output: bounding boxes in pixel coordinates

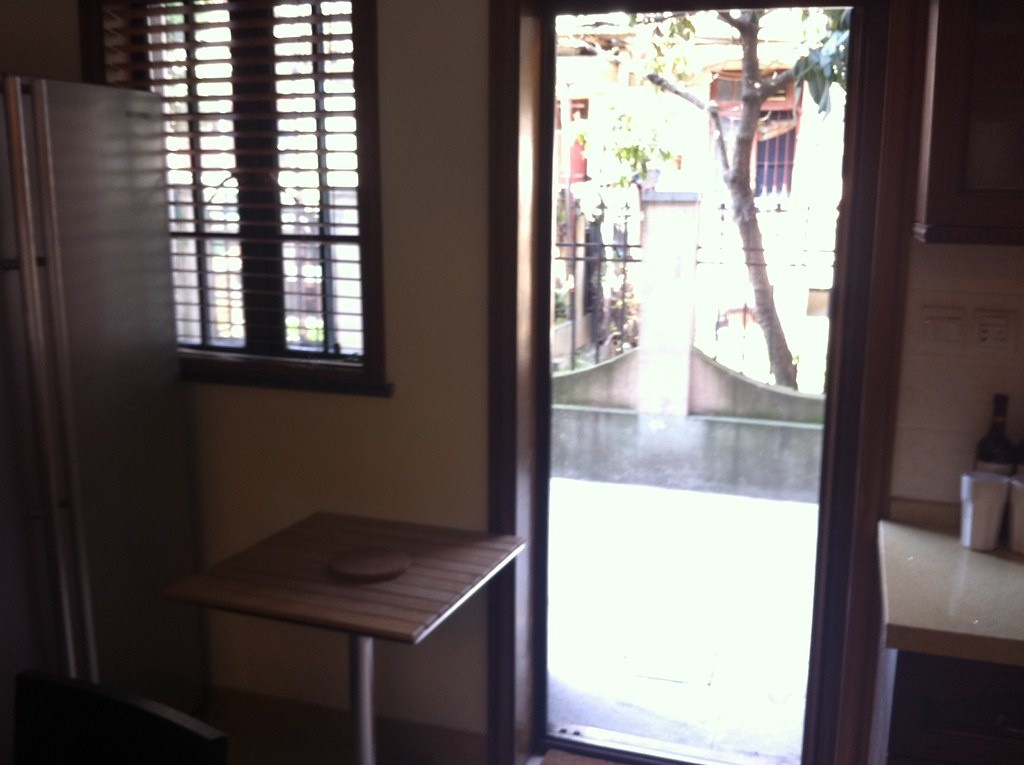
[971,308,1015,357]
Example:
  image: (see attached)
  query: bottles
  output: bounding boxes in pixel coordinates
[1017,432,1024,482]
[973,387,1016,476]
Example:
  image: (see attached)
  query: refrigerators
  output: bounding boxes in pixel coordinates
[0,70,210,765]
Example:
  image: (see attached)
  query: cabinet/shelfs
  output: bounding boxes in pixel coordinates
[869,613,1024,765]
[911,0,1024,245]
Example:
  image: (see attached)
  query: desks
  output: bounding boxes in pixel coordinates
[158,511,529,764]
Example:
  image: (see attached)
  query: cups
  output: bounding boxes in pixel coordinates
[959,471,1011,554]
[1009,479,1024,554]
